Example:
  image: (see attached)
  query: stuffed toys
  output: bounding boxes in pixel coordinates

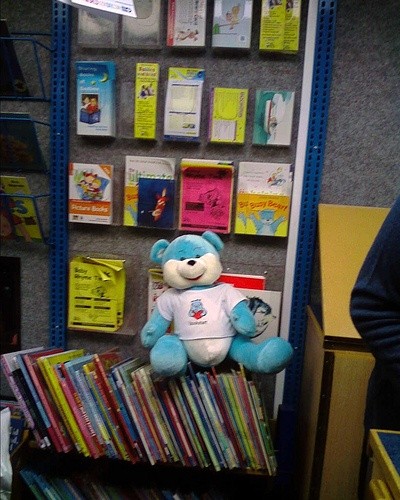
[140,230,295,380]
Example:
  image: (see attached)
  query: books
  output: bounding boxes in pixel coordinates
[210,1,254,51]
[0,345,278,477]
[68,162,114,226]
[133,61,160,140]
[166,0,208,48]
[233,288,282,344]
[207,88,249,145]
[178,157,235,235]
[147,268,175,335]
[67,256,127,333]
[12,462,288,500]
[251,88,295,147]
[234,161,294,238]
[217,273,267,290]
[74,59,116,138]
[162,67,206,141]
[259,0,303,54]
[1,176,46,242]
[123,154,177,230]
[0,109,51,174]
[1,19,33,100]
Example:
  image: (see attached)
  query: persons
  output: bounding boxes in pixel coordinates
[347,190,400,499]
